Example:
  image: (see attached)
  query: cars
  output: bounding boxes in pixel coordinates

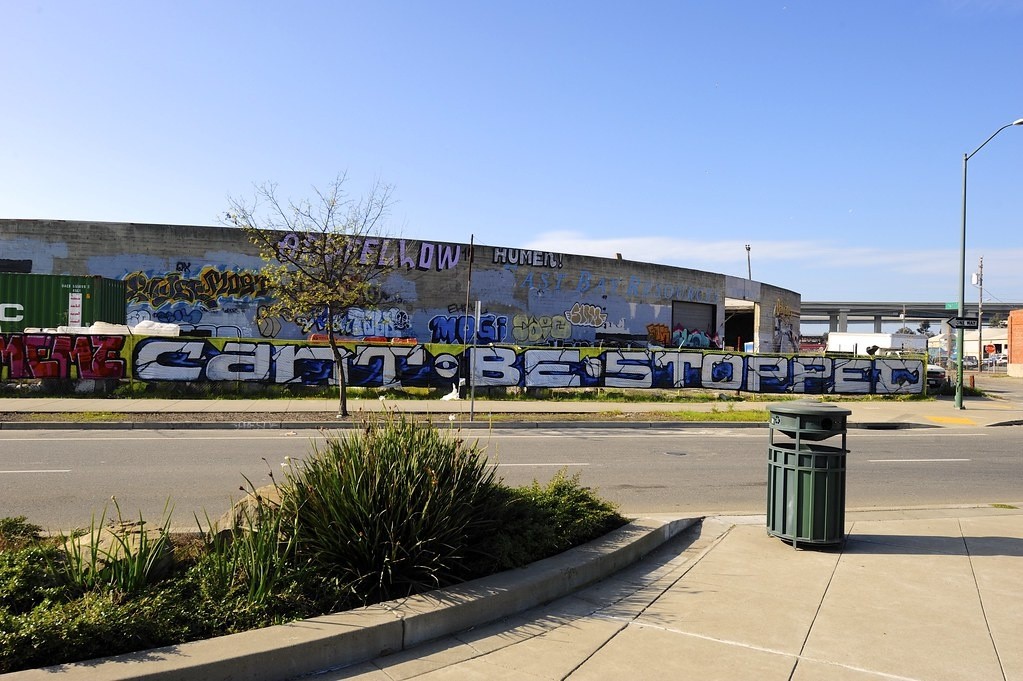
[961,354,1007,370]
[927,359,946,388]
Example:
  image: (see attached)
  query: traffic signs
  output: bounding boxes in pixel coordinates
[946,317,979,330]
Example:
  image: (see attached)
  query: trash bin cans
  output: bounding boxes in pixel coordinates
[764,400,852,554]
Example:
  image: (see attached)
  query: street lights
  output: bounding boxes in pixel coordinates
[954,118,1023,411]
